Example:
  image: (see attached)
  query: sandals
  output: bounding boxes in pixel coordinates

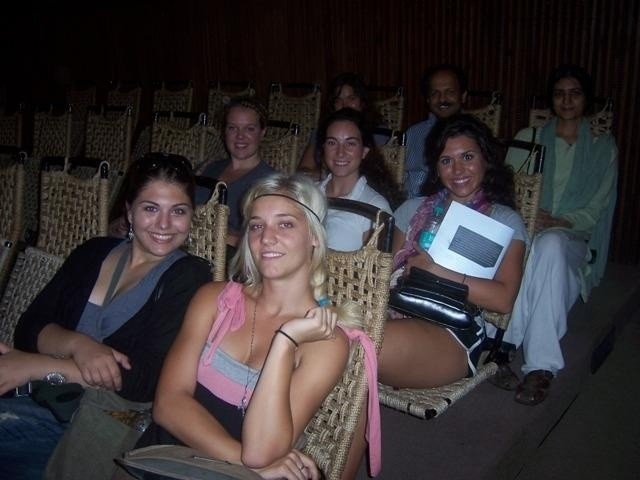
[486,363,553,405]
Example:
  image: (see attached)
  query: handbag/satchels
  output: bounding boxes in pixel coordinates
[42,388,153,480]
[387,266,474,330]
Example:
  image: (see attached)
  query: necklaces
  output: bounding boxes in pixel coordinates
[236,297,261,417]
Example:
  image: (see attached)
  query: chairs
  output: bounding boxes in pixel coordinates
[1,77,615,480]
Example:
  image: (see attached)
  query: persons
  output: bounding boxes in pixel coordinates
[308,107,394,254]
[0,149,215,480]
[295,70,388,182]
[339,112,532,478]
[399,61,470,199]
[108,172,354,479]
[499,63,621,407]
[108,93,276,280]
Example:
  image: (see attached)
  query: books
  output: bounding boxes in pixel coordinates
[427,200,516,280]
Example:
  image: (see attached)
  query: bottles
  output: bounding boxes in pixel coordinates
[418,206,445,252]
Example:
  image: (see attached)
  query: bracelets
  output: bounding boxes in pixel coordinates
[461,273,467,284]
[44,350,66,385]
[273,329,300,349]
